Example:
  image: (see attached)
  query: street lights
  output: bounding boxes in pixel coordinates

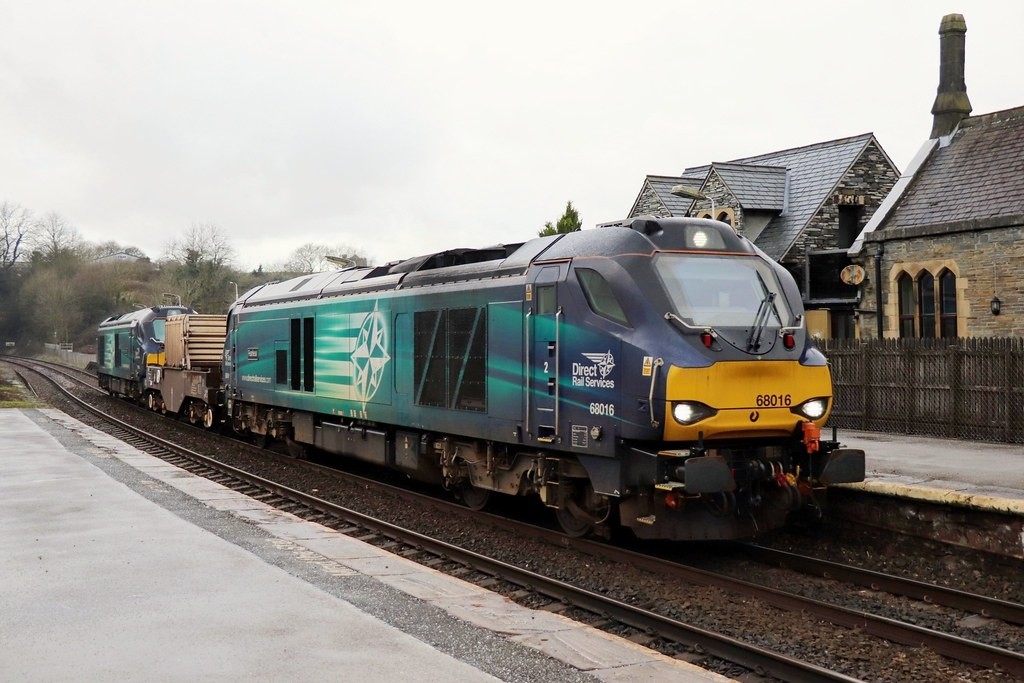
[672,186,715,219]
[324,254,356,268]
[226,280,238,299]
[163,293,181,307]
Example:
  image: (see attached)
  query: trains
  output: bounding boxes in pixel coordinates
[93,213,865,539]
